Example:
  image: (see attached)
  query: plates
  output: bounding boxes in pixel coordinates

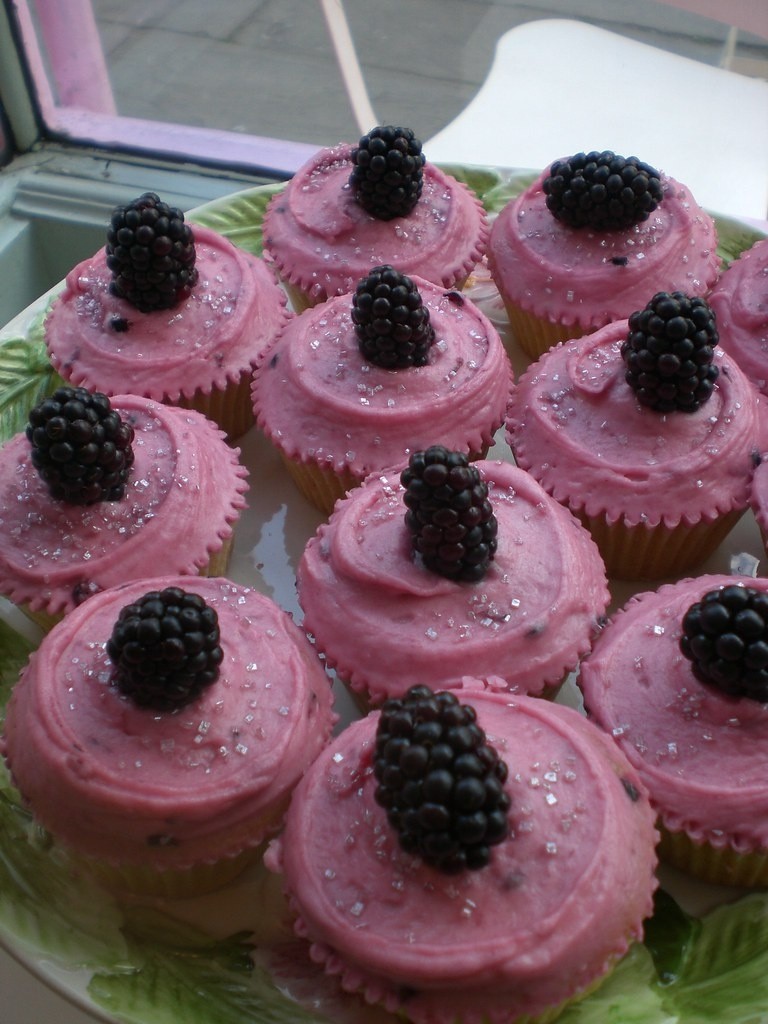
[1,166,767,1023]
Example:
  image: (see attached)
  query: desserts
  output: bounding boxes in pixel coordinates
[1,120,768,1024]
